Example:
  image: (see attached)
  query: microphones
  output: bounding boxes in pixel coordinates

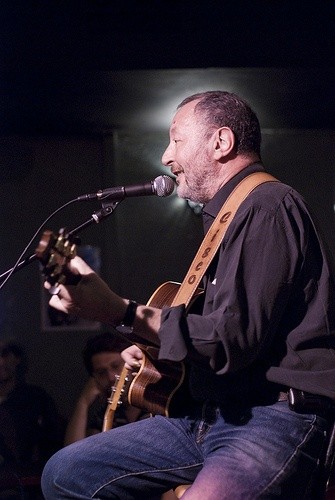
[77,175,175,200]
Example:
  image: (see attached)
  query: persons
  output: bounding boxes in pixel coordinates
[0,337,58,500]
[39,91,335,500]
[65,328,150,447]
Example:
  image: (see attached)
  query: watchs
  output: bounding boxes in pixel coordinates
[116,299,137,335]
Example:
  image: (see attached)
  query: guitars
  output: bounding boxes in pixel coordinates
[102,359,129,431]
[29,227,207,419]
